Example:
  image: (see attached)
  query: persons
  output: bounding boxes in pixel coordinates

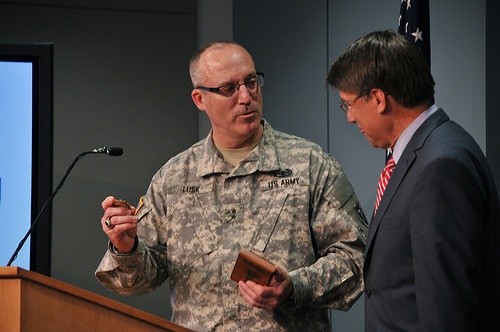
[325,29,500,332]
[94,39,371,332]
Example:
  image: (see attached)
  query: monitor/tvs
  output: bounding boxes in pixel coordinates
[0,42,55,276]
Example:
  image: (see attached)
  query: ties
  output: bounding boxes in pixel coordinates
[373,156,395,216]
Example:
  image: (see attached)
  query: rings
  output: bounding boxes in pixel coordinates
[104,216,112,228]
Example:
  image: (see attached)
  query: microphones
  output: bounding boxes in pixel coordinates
[7,147,123,267]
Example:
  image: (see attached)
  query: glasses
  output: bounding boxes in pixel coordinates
[196,72,266,97]
[338,90,371,113]
